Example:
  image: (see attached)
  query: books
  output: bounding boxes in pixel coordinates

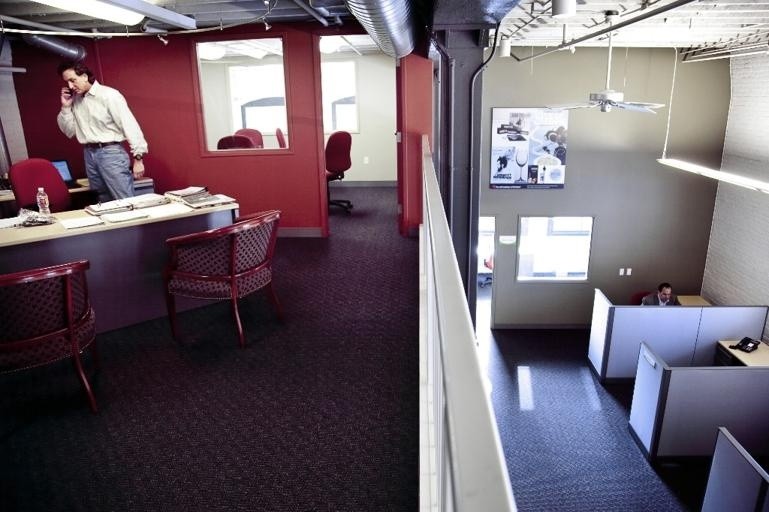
[74,178,90,187]
[0,208,55,229]
[0,187,16,202]
[60,183,238,230]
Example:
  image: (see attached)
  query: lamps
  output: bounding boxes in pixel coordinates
[498,0,577,57]
[655,48,769,195]
[155,17,275,49]
[31,0,197,31]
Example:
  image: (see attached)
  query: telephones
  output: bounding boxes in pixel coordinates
[736,336,761,353]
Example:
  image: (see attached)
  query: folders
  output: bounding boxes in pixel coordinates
[166,186,208,198]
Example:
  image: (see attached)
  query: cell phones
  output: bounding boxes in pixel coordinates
[67,86,73,96]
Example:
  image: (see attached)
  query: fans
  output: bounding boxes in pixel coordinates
[542,10,668,119]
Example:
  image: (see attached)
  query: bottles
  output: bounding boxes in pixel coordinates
[36,186,51,218]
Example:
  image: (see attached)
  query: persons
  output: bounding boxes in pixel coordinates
[55,58,149,201]
[496,153,507,172]
[638,282,682,305]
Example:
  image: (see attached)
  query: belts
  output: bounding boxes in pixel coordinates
[81,141,121,150]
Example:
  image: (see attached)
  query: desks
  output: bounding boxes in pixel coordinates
[0,194,240,334]
[714,339,769,366]
[0,176,155,218]
[674,295,712,306]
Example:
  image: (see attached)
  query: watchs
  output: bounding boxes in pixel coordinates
[133,154,143,160]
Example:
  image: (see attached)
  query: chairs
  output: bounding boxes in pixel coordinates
[163,209,281,350]
[8,158,72,215]
[629,289,652,305]
[1,255,105,418]
[326,130,353,216]
[217,126,285,150]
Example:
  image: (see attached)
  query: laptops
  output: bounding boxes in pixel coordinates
[48,159,82,189]
